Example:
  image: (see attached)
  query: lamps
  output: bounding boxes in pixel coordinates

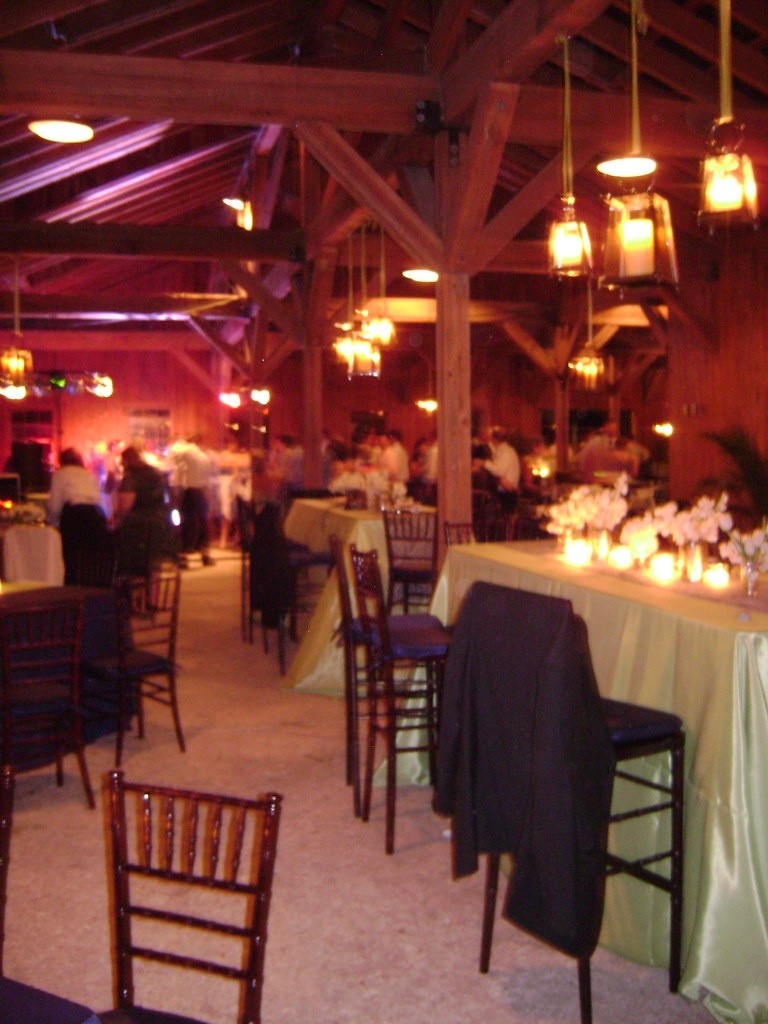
[332,218,396,377]
[0,259,27,400]
[696,0,768,228]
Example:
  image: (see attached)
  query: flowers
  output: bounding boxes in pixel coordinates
[644,492,733,546]
[545,472,629,537]
[719,516,768,579]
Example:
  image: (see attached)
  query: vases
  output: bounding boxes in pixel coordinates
[679,541,709,583]
[740,561,761,599]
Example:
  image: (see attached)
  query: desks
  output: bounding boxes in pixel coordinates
[1,520,65,586]
[275,498,478,702]
[374,538,768,1024]
[0,586,139,774]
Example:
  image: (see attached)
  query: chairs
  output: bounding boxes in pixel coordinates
[547,0,681,378]
[96,770,283,1024]
[0,549,187,977]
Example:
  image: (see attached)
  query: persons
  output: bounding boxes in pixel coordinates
[117,447,167,565]
[165,432,520,630]
[45,447,109,591]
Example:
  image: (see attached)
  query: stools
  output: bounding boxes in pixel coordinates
[232,493,687,1024]
